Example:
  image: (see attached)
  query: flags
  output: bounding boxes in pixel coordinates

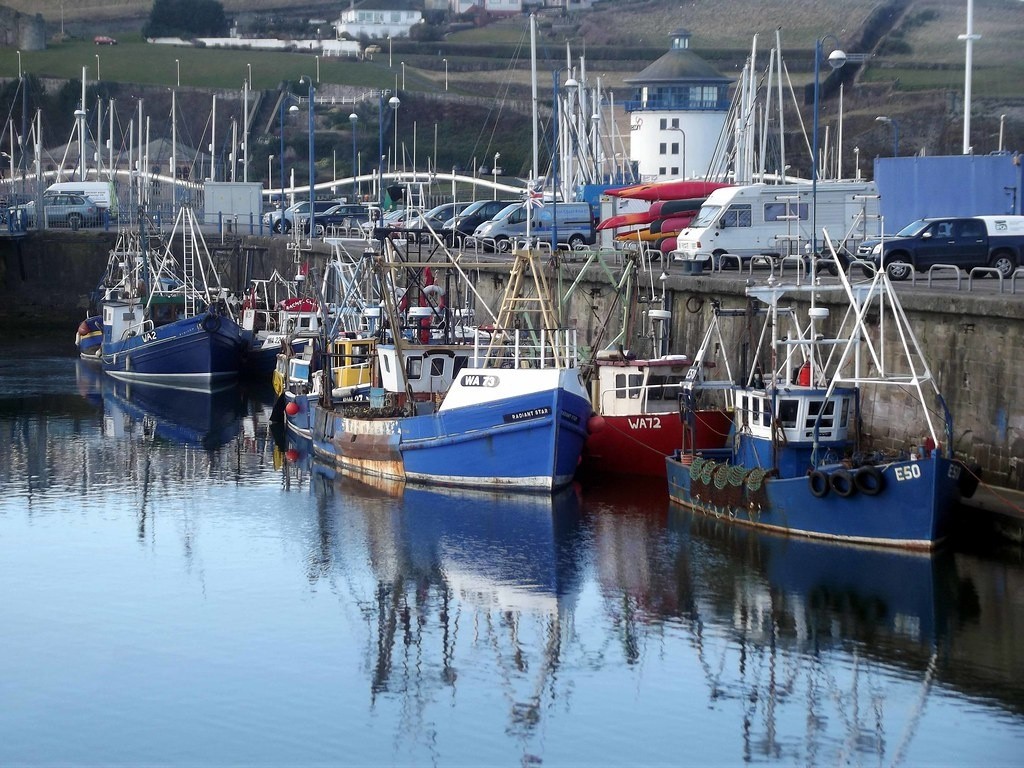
[522,185,544,210]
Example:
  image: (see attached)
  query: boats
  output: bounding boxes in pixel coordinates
[665,225,985,553]
[572,229,741,487]
[73,166,508,388]
[662,499,940,767]
[572,466,734,669]
[273,229,596,494]
[272,438,598,768]
[95,368,283,550]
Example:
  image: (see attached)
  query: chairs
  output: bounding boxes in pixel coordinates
[520,209,527,219]
[937,225,947,237]
[48,197,53,205]
[949,227,960,238]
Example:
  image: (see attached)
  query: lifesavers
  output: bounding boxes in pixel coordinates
[420,285,445,312]
[854,465,883,495]
[828,469,855,497]
[808,471,829,497]
[383,286,407,314]
[202,313,222,333]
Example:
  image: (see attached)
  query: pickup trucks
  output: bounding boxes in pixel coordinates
[853,217,1024,282]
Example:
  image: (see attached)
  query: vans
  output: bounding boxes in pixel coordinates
[440,199,523,248]
[472,202,593,254]
[27,181,115,220]
[672,182,881,271]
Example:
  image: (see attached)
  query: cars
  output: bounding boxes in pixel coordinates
[401,201,475,244]
[94,36,118,47]
[359,207,430,238]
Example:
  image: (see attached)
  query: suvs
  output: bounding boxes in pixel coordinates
[262,199,348,236]
[6,192,100,231]
[298,202,382,235]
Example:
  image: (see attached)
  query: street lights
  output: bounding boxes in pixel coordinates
[809,32,849,258]
[589,114,602,183]
[378,86,402,228]
[552,65,578,265]
[350,106,361,204]
[300,74,316,238]
[280,97,301,236]
[875,117,899,158]
